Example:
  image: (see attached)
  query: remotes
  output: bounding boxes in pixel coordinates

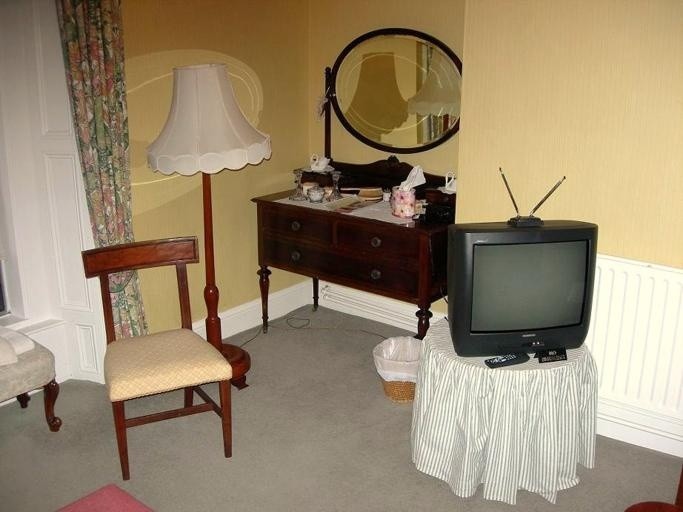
[484,351,530,368]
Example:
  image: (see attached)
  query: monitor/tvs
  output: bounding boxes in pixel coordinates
[447,219,599,357]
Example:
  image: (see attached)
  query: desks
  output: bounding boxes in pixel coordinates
[411,315,598,505]
[249,189,449,340]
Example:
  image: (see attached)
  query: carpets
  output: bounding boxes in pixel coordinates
[52,483,155,511]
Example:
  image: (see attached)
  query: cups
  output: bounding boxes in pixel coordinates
[382,192,392,202]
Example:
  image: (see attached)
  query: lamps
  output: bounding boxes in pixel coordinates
[145,61,273,390]
[404,49,462,134]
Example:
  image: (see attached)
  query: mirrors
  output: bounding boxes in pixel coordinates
[327,25,462,153]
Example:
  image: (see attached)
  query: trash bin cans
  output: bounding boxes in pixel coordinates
[373,336,422,404]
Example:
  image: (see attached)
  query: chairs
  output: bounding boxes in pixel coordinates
[80,234,232,481]
